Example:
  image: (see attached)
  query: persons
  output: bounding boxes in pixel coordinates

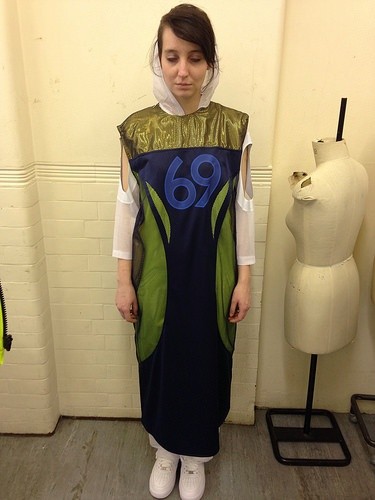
[282,138,368,354]
[110,3,258,500]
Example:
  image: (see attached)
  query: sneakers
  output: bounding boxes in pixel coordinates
[178,461,206,500]
[148,449,179,499]
